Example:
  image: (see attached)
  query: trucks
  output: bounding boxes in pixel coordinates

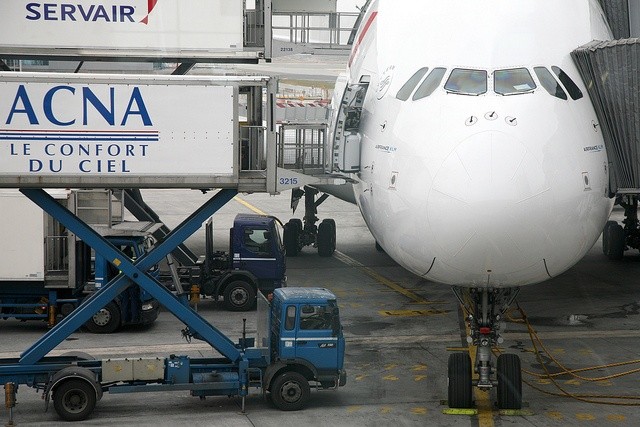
[2,186,164,335]
[87,212,290,311]
[2,286,348,421]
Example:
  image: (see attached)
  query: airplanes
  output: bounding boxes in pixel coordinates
[277,1,640,410]
